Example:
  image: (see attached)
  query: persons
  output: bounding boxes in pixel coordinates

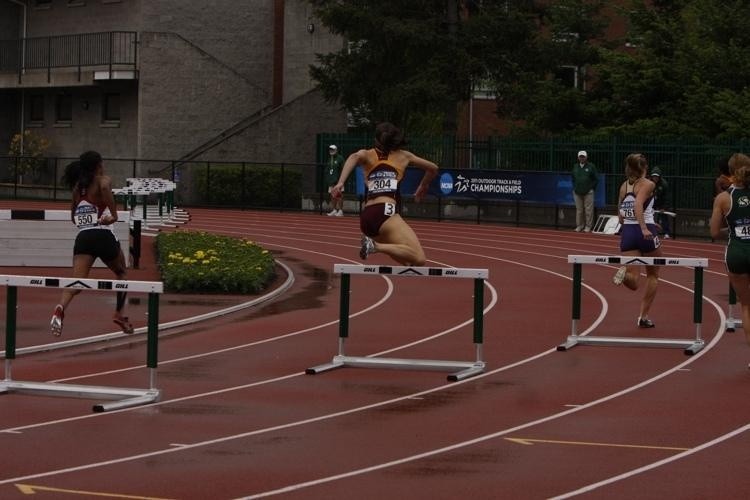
[710,152,750,343]
[611,152,664,329]
[713,174,734,192]
[571,150,602,232]
[325,144,347,217]
[49,150,136,338]
[645,165,672,240]
[329,121,440,267]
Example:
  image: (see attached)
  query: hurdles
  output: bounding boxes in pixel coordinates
[557,254,708,354]
[726,279,742,331]
[0,178,191,269]
[0,275,164,412]
[306,264,489,381]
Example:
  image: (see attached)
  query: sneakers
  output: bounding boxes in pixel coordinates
[326,208,344,218]
[48,303,65,338]
[111,310,133,334]
[574,225,582,232]
[359,235,375,261]
[584,225,591,233]
[637,316,655,328]
[612,265,628,286]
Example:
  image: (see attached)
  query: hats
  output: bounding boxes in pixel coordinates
[329,144,337,152]
[576,150,587,162]
